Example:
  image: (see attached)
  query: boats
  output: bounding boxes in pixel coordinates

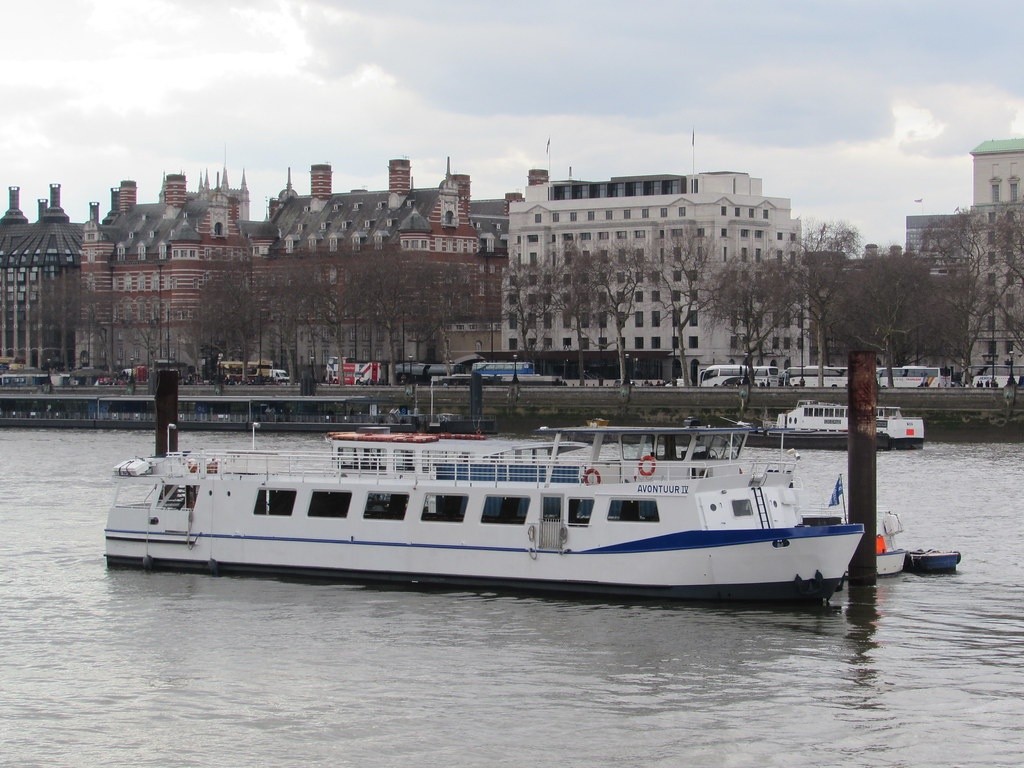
[725,399,924,450]
[106,370,864,603]
[907,551,963,572]
[877,513,905,575]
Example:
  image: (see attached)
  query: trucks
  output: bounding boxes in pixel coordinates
[327,363,387,385]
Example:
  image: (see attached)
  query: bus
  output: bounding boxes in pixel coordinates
[782,365,848,387]
[960,365,1024,388]
[876,366,951,387]
[472,363,536,376]
[699,365,779,388]
[1,374,71,387]
[219,361,274,381]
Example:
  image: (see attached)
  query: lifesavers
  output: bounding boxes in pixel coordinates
[638,455,657,476]
[584,468,601,485]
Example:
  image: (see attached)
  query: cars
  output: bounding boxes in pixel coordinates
[276,369,290,383]
